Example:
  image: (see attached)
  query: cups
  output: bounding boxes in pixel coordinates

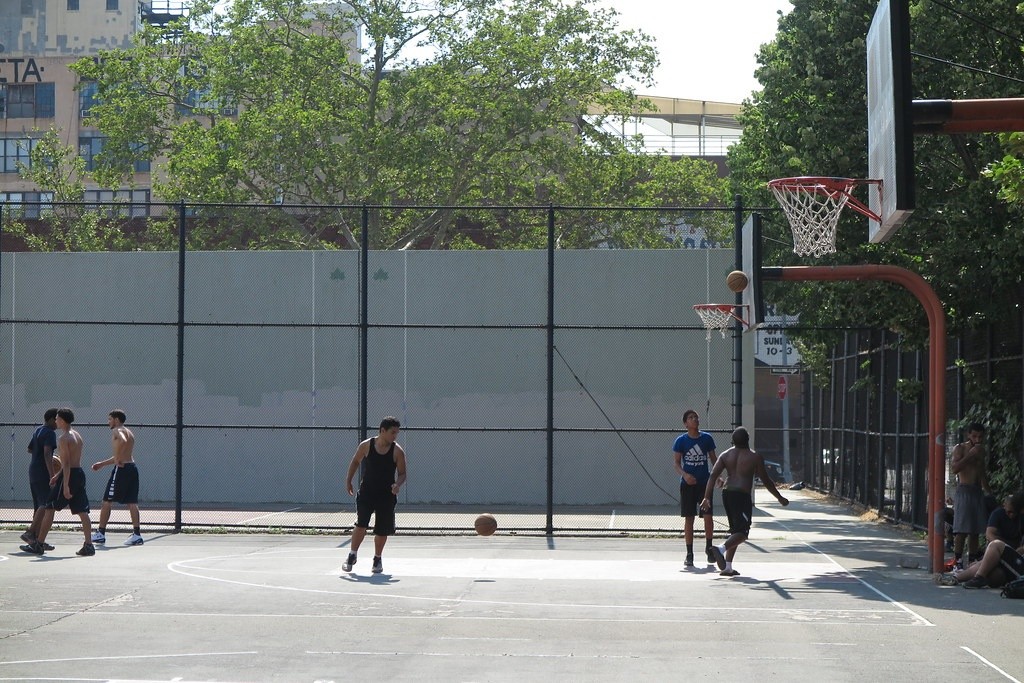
[975,444,981,446]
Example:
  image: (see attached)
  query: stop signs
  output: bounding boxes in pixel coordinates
[776,376,787,400]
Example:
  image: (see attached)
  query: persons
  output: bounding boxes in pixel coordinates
[945,496,954,551]
[951,422,994,571]
[699,426,789,576]
[931,486,1024,589]
[672,409,723,566]
[91,409,144,544]
[986,494,1024,550]
[20,408,57,550]
[342,416,406,573]
[19,408,95,555]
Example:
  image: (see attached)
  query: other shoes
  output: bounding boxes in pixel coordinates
[709,545,727,570]
[720,570,739,575]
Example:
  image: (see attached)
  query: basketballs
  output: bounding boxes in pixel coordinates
[727,270,750,292]
[474,513,498,537]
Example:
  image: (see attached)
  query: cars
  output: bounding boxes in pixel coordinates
[755,458,782,485]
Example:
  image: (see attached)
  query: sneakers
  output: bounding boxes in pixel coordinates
[684,553,694,565]
[124,534,144,545]
[937,573,959,585]
[44,542,55,550]
[342,553,357,571]
[953,558,964,573]
[20,530,37,544]
[962,578,982,589]
[706,549,716,563]
[76,543,95,556]
[91,529,105,543]
[372,556,383,572]
[19,539,44,555]
